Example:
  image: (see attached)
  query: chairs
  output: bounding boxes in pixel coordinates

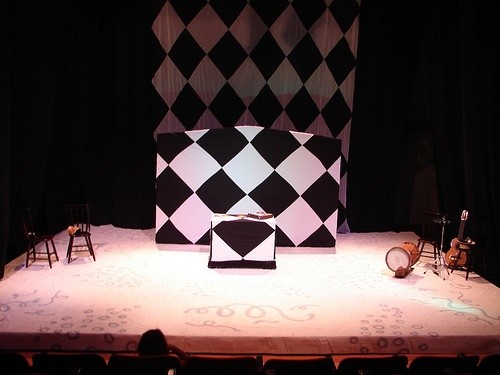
[0,350,500,375]
[67,204,95,263]
[22,208,59,268]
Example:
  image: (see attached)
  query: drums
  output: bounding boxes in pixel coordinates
[385,241,420,272]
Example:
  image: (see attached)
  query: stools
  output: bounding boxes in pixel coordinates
[417,213,449,268]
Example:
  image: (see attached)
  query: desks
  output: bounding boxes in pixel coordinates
[208,213,276,270]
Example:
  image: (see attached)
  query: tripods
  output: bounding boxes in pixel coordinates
[424,222,452,280]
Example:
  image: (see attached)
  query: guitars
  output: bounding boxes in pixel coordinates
[445,210,469,267]
[214,212,273,219]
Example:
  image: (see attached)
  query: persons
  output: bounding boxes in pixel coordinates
[136,329,189,369]
